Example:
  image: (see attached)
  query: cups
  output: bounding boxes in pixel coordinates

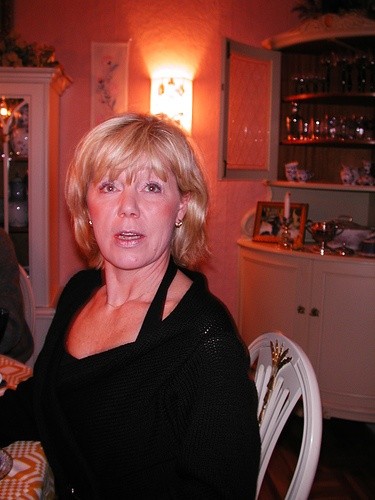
[297,169,308,181]
[284,161,298,180]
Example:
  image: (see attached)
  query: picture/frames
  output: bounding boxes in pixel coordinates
[252,198,310,251]
[91,41,130,128]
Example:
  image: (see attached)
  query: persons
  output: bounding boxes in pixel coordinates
[0,113,262,499]
[0,227,35,365]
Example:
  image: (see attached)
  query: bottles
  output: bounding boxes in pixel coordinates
[285,102,367,140]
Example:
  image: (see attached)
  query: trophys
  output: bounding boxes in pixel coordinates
[305,219,345,255]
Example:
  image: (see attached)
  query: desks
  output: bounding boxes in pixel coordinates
[0,355,54,500]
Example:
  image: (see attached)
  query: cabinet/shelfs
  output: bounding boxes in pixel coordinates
[218,16,375,425]
[0,67,76,367]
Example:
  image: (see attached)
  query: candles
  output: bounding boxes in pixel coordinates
[283,191,291,220]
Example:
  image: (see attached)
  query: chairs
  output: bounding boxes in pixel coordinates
[240,332,324,500]
